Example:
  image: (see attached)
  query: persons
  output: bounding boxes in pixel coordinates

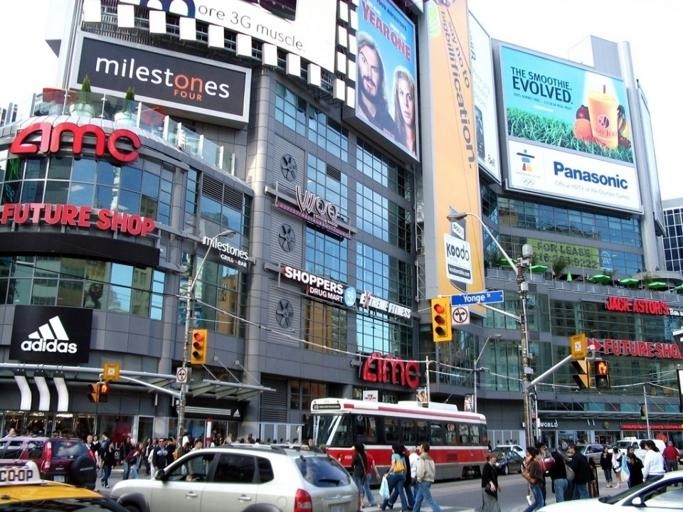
[351,441,440,511]
[119,429,329,482]
[482,435,682,512]
[336,430,487,448]
[3,428,116,488]
[393,69,416,156]
[357,32,394,136]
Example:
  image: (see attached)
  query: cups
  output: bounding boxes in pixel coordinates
[587,92,631,149]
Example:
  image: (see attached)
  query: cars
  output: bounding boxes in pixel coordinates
[0,459,132,512]
[489,437,666,476]
[524,468,682,510]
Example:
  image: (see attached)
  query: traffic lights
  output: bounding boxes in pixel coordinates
[593,359,611,391]
[87,383,96,402]
[98,384,109,403]
[189,327,208,369]
[572,358,590,391]
[429,297,451,343]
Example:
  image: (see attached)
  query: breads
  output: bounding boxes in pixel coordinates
[574,119,593,140]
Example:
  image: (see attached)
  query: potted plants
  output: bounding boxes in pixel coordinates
[114,85,140,142]
[69,72,96,119]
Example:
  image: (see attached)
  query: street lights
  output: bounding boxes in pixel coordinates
[471,333,503,414]
[177,228,234,453]
[443,213,541,503]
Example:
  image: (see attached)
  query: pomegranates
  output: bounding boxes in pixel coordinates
[576,104,589,121]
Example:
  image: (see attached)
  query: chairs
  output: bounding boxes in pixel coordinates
[384,425,480,446]
[214,463,241,482]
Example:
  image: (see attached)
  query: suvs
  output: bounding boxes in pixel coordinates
[0,434,97,493]
[108,444,359,512]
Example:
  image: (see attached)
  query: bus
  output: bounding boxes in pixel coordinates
[306,392,491,489]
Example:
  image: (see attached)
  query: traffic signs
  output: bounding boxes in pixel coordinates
[451,290,504,306]
